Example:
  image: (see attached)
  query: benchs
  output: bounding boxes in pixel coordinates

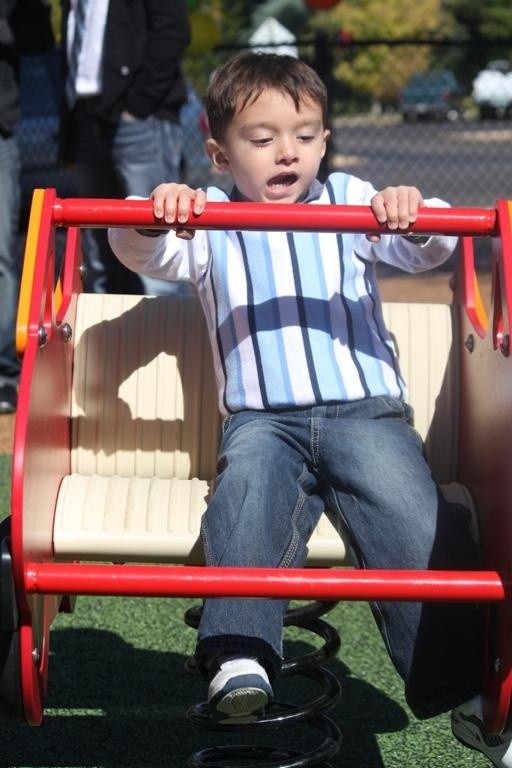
[52,293,483,568]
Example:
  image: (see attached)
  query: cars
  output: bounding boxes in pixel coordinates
[399,70,458,121]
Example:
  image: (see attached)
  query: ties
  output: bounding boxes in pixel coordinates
[65,0,84,112]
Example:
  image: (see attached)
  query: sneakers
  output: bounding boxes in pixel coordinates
[451,695,511,767]
[206,655,274,725]
[0,377,19,412]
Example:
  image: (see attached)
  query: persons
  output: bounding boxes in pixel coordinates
[0,2,210,415]
[109,49,512,766]
